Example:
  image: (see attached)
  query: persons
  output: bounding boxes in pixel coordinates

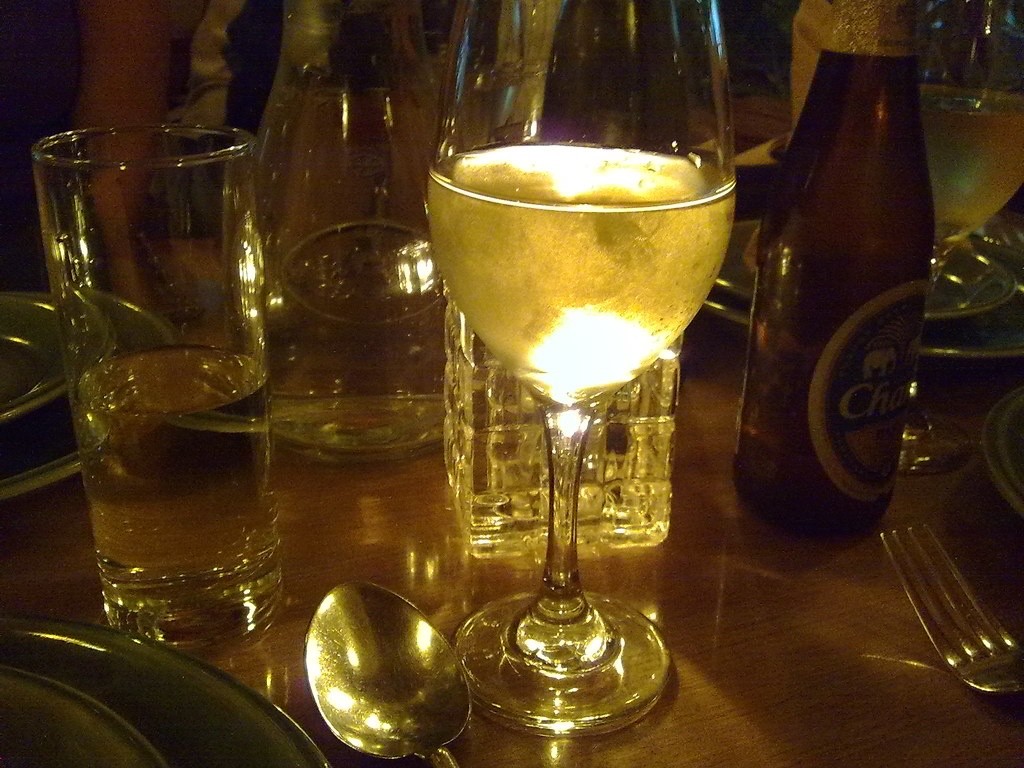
[79,0,436,308]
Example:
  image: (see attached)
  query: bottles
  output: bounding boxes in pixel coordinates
[446,227,461,241]
[731,0,937,545]
[251,0,461,467]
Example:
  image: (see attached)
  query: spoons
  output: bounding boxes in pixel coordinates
[300,578,461,768]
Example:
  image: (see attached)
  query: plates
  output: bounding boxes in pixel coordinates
[0,613,329,768]
[0,289,175,504]
[702,218,1024,363]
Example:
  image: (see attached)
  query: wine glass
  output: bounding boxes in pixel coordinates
[423,1,737,736]
[876,0,1024,477]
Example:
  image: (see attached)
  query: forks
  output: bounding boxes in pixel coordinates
[882,522,1024,699]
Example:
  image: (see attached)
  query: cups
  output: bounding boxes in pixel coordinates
[33,124,287,652]
